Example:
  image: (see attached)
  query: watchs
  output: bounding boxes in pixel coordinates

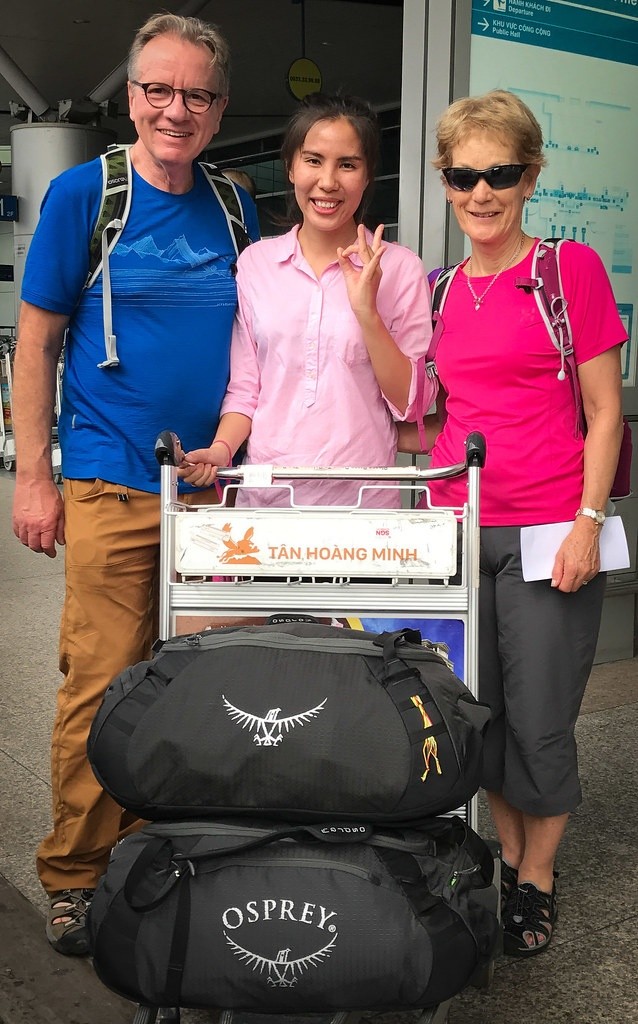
[574,507,606,526]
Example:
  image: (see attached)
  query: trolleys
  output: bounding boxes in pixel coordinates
[149,429,503,1016]
[0,333,69,483]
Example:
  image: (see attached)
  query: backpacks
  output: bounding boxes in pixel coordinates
[418,238,634,504]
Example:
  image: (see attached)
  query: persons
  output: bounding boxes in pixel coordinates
[395,87,629,960]
[13,13,265,961]
[175,91,437,586]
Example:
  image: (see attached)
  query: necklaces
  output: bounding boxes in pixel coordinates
[465,231,525,312]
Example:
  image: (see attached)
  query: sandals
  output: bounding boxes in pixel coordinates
[46,888,96,954]
[500,870,559,957]
[500,857,518,916]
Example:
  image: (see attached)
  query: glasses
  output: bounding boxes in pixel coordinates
[441,162,529,193]
[130,79,223,115]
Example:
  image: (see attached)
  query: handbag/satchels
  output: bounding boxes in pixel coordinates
[84,814,495,1014]
[85,622,492,827]
